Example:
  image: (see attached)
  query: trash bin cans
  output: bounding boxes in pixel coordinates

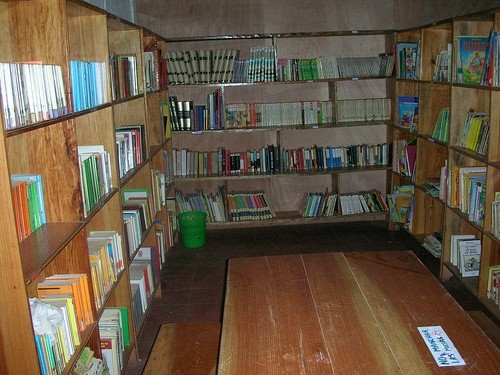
[176,211,208,249]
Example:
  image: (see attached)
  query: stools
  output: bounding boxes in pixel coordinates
[140,321,221,375]
[466,311,500,352]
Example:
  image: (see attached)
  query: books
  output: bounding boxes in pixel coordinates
[0,33,500,375]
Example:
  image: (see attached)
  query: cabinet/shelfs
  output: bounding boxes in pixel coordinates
[0,0,181,375]
[390,6,500,321]
[166,28,393,229]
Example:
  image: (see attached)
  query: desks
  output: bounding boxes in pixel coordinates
[216,250,500,375]
